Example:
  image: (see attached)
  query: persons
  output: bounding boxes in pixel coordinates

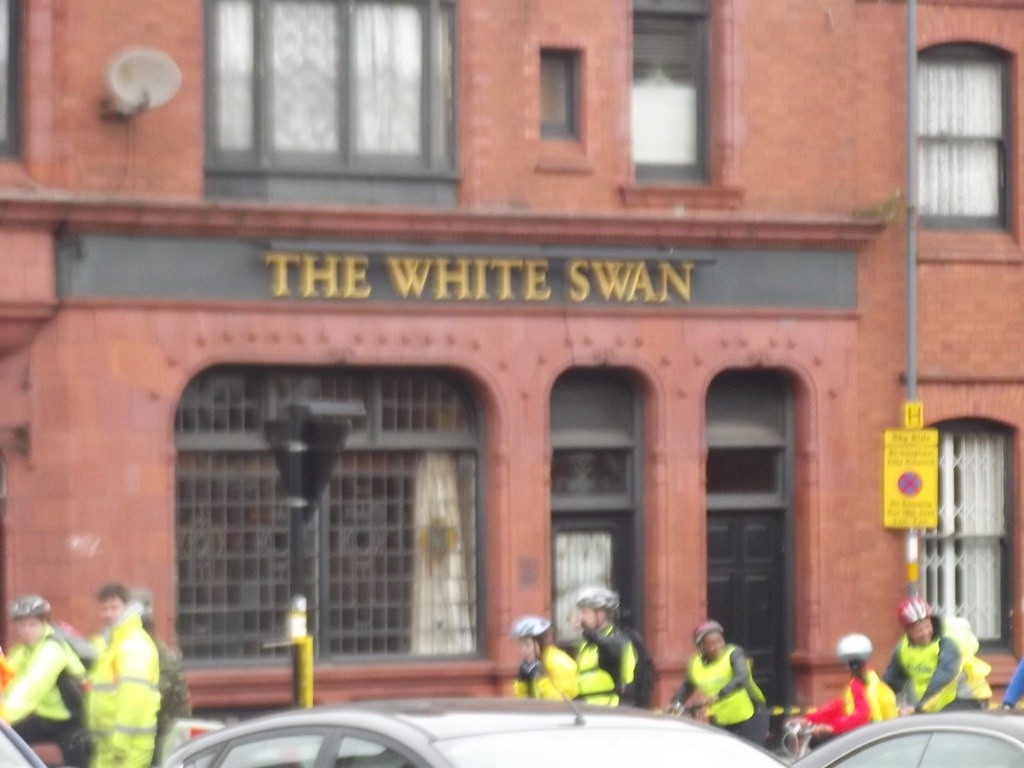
[513,586,1024,750]
[0,582,192,768]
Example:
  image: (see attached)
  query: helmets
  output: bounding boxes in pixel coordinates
[693,619,728,652]
[512,616,553,639]
[10,596,51,619]
[836,635,873,662]
[576,586,620,611]
[895,598,935,627]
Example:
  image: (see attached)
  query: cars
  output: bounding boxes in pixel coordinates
[796,708,1024,767]
[156,695,783,768]
